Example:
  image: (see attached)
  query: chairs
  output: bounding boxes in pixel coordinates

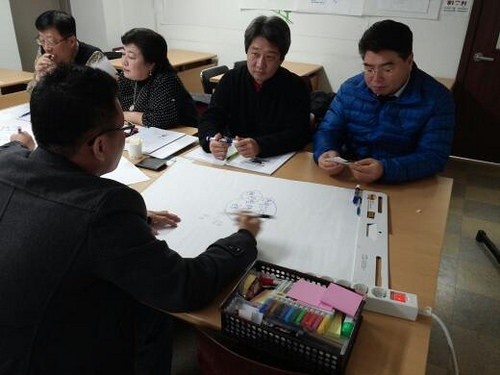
[177,63,217,94]
[199,65,229,94]
[0,90,30,110]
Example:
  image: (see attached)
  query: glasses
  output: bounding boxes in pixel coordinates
[87,120,135,146]
[35,35,72,48]
[362,65,394,76]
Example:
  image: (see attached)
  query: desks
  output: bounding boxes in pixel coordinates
[209,59,325,80]
[0,65,34,89]
[122,125,199,164]
[109,48,218,75]
[125,151,455,375]
[432,76,456,91]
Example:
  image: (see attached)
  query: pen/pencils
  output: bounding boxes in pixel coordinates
[206,137,240,142]
[18,126,21,131]
[358,197,360,214]
[48,54,52,59]
[226,210,271,218]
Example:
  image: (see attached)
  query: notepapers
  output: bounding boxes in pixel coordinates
[288,277,332,311]
[320,282,362,317]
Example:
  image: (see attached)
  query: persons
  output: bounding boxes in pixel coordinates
[197,16,312,159]
[26,10,119,97]
[0,131,34,153]
[313,20,454,185]
[0,64,259,375]
[116,28,198,129]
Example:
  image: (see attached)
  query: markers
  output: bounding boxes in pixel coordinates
[259,278,336,334]
[353,183,361,203]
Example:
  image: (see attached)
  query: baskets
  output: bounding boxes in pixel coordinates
[221,259,365,375]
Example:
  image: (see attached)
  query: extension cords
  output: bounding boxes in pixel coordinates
[304,272,418,321]
